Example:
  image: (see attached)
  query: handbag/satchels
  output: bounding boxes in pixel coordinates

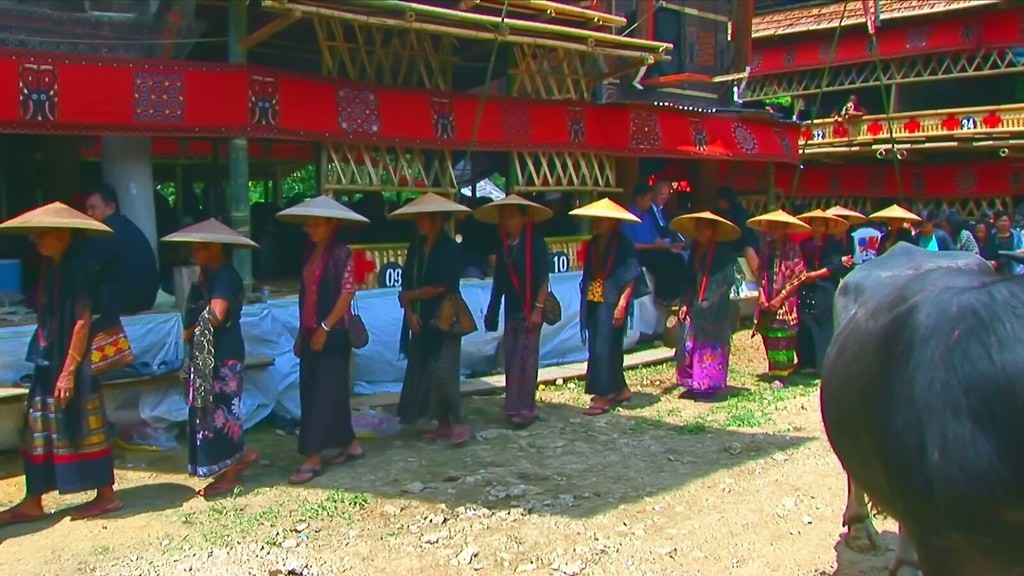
[348,315,369,349]
[428,290,478,335]
[586,279,604,303]
[544,291,561,325]
[90,313,134,376]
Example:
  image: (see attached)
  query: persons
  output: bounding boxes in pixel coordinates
[160,218,262,496]
[567,197,643,415]
[795,204,1024,380]
[273,193,371,484]
[0,183,577,314]
[746,208,813,387]
[473,193,554,424]
[0,200,124,521]
[841,95,866,119]
[669,210,742,393]
[388,192,475,445]
[620,180,691,309]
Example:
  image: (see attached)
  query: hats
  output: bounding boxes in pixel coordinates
[0,201,114,236]
[474,194,554,224]
[389,193,473,220]
[275,194,371,226]
[568,197,642,224]
[669,210,742,241]
[746,204,922,234]
[160,218,260,249]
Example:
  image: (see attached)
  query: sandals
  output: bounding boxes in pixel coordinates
[420,424,472,443]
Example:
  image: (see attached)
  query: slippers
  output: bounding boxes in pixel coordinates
[233,452,260,469]
[289,467,323,485]
[198,477,240,496]
[583,391,632,415]
[0,506,47,527]
[70,501,124,519]
[331,449,366,466]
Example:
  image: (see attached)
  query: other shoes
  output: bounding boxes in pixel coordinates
[506,412,539,425]
[679,390,725,402]
[770,378,790,388]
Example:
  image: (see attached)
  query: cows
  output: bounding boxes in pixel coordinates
[819,241,1024,576]
